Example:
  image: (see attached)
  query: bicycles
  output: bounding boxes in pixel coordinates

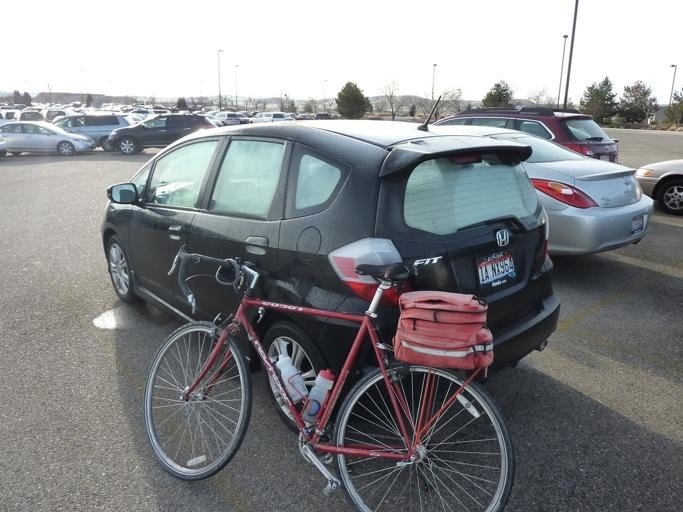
[143,241,515,512]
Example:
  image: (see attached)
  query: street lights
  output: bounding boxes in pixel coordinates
[557,34,569,108]
[432,63,438,100]
[667,64,677,106]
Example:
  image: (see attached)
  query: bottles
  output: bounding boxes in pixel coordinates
[275,353,308,405]
[297,367,336,428]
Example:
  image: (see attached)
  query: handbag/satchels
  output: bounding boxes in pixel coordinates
[392,289,494,370]
